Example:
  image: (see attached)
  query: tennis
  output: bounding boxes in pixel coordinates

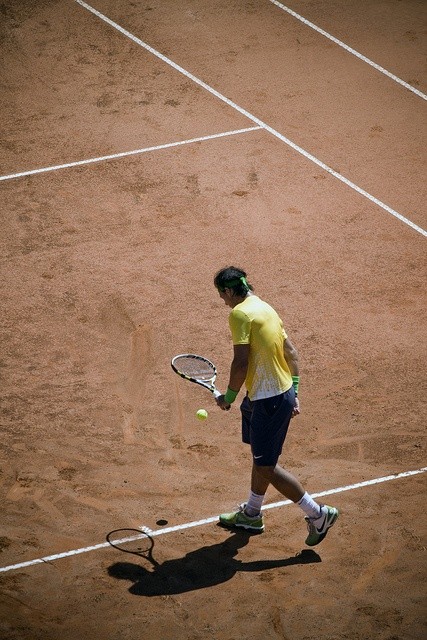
[196,410,207,420]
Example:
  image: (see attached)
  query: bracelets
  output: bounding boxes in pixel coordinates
[222,386,240,403]
[292,375,299,396]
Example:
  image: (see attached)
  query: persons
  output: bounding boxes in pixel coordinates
[214,266,339,546]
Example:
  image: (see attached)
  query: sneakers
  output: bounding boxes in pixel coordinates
[304,505,339,546]
[219,503,264,533]
